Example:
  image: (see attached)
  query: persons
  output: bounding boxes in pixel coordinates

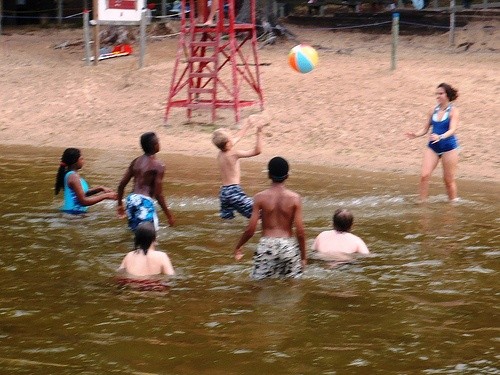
[116,132,175,230]
[406,83,460,197]
[119,221,174,273]
[211,122,271,219]
[232,157,307,279]
[54,148,118,210]
[312,210,370,253]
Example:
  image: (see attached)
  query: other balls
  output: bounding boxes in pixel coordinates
[288,44,318,73]
